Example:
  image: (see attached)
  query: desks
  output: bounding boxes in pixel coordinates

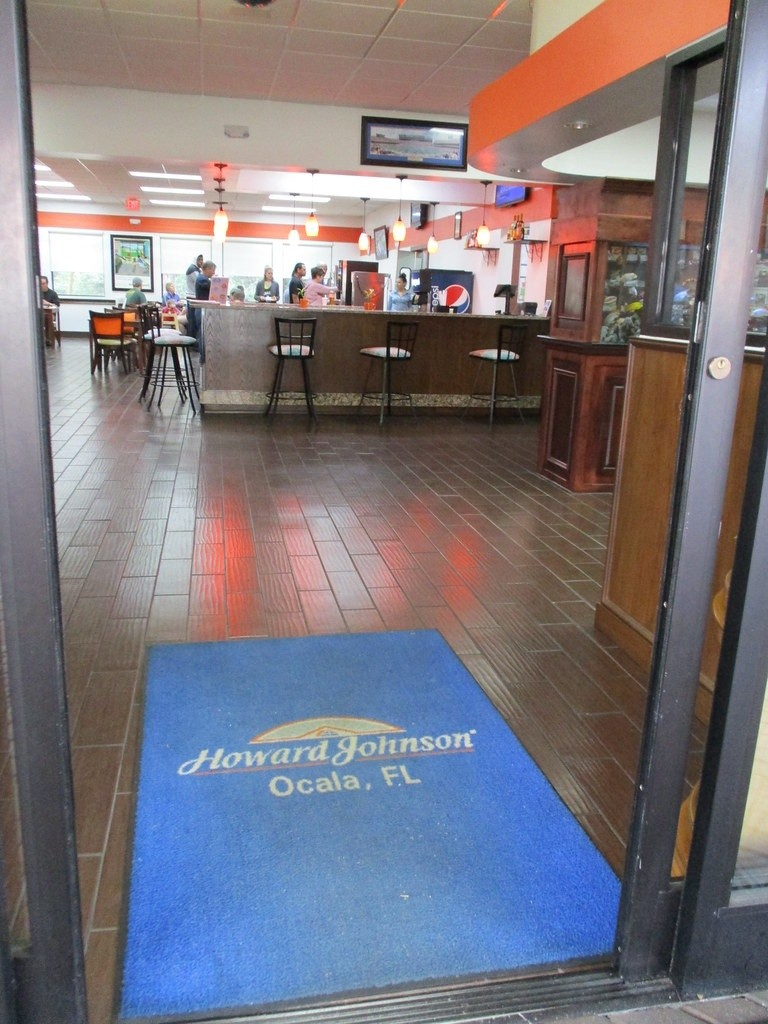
[43,306,61,348]
[88,319,146,376]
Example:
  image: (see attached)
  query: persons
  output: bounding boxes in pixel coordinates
[229,285,245,302]
[40,275,59,345]
[289,262,306,304]
[387,274,412,312]
[126,277,147,307]
[162,281,180,305]
[316,263,327,285]
[303,266,338,305]
[162,301,180,321]
[254,267,279,303]
[178,255,217,352]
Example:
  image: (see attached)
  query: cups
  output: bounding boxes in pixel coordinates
[364,303,376,310]
[449,308,454,314]
[300,299,309,308]
[322,298,328,306]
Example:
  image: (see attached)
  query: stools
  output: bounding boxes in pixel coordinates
[355,321,419,426]
[461,325,528,430]
[137,301,199,414]
[264,317,319,426]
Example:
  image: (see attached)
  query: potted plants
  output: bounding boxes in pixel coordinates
[297,288,308,308]
[363,288,375,311]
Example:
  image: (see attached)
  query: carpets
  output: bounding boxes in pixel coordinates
[109,627,623,1023]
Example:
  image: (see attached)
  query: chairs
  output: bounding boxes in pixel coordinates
[87,304,142,374]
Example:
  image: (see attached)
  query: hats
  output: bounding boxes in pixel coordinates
[133,277,141,287]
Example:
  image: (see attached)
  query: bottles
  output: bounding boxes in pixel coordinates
[329,291,335,305]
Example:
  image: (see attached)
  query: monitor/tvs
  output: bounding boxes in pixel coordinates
[373,225,388,260]
[410,203,427,228]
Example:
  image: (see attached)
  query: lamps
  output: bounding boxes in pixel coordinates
[359,198,370,250]
[289,193,300,245]
[393,175,407,242]
[427,202,439,255]
[477,181,492,245]
[213,164,228,239]
[305,169,319,237]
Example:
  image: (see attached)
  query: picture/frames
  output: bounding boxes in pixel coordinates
[360,116,468,172]
[111,235,154,292]
